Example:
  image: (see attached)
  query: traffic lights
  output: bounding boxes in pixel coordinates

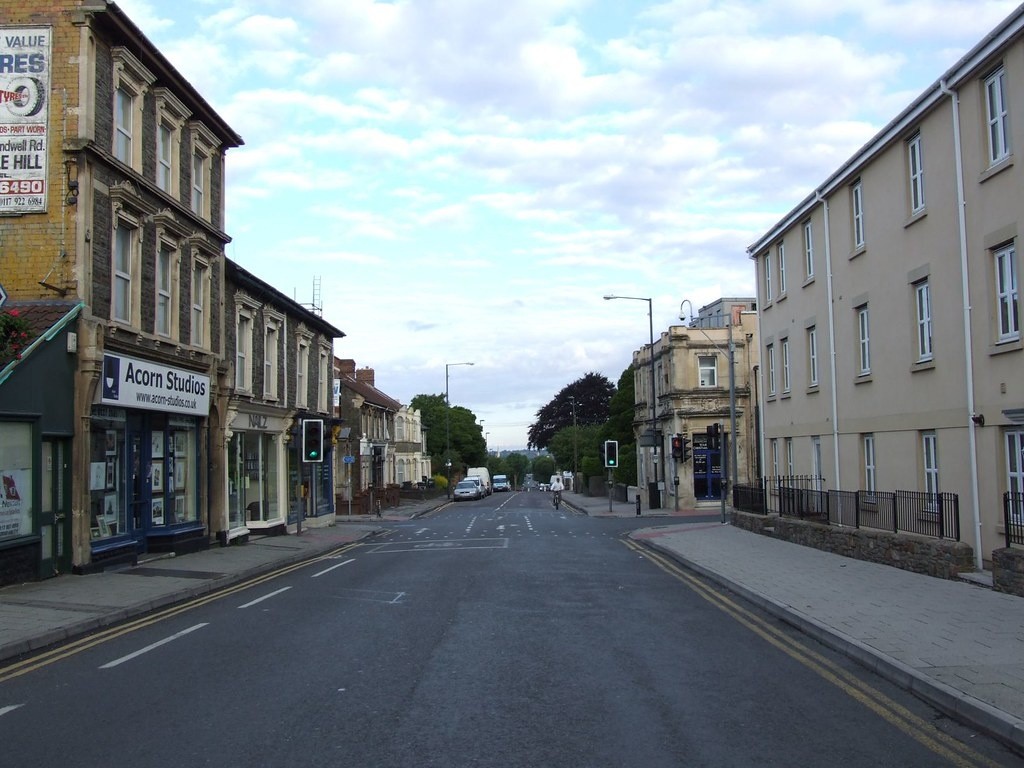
[605,441,619,468]
[671,437,682,459]
[303,419,323,463]
[707,423,718,451]
[681,437,692,464]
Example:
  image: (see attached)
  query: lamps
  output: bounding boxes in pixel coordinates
[63,156,80,206]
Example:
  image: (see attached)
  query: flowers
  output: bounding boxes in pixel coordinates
[0,309,33,363]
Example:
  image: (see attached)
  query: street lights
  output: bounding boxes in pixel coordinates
[567,396,578,494]
[446,362,474,499]
[602,294,658,483]
[679,300,738,486]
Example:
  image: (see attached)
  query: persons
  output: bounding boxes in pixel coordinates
[550,478,564,506]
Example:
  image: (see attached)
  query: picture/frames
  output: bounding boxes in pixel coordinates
[90,429,119,539]
[151,429,188,524]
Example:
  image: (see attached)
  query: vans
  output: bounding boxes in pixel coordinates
[467,467,492,496]
[492,475,508,492]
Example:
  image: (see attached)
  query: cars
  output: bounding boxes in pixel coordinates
[453,481,480,501]
[506,481,513,492]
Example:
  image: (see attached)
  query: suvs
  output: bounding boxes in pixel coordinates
[462,475,486,500]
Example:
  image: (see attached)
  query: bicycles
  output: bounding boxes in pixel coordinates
[551,489,564,510]
